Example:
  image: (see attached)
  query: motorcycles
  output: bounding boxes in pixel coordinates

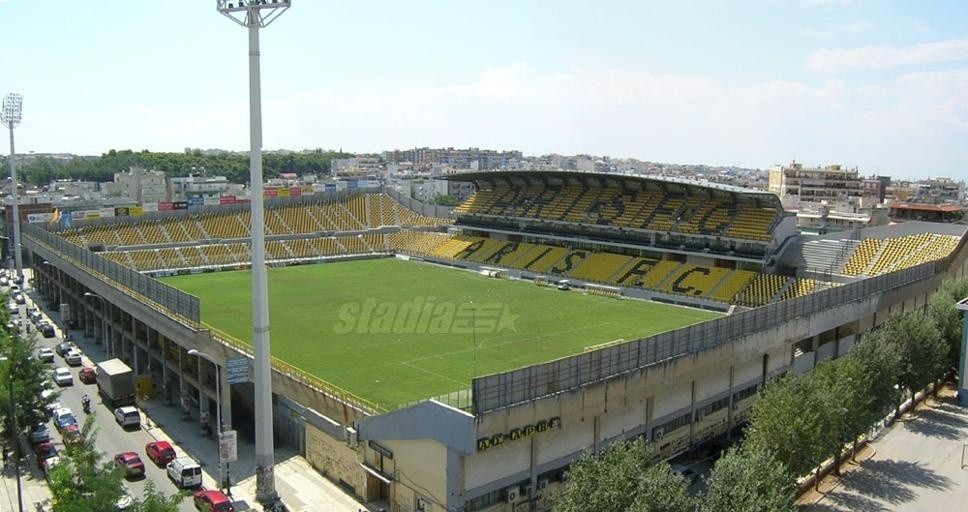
[83,398,91,413]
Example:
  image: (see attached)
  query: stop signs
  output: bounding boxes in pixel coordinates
[142,393,149,401]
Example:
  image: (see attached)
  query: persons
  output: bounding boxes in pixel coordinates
[26,321,30,330]
[82,395,90,407]
[2,447,8,466]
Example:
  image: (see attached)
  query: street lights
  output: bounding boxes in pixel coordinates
[0,93,22,276]
[188,348,222,489]
[217,1,292,503]
[44,260,62,304]
[1,234,34,303]
[85,292,110,361]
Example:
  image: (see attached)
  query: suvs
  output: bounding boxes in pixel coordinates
[113,406,141,429]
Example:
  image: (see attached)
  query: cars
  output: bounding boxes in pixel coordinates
[27,388,82,489]
[39,343,95,387]
[0,269,55,338]
[115,452,145,478]
[194,490,233,511]
[146,441,176,468]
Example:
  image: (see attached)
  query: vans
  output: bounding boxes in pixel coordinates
[167,457,203,490]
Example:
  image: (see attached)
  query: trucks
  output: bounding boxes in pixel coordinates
[94,357,136,407]
